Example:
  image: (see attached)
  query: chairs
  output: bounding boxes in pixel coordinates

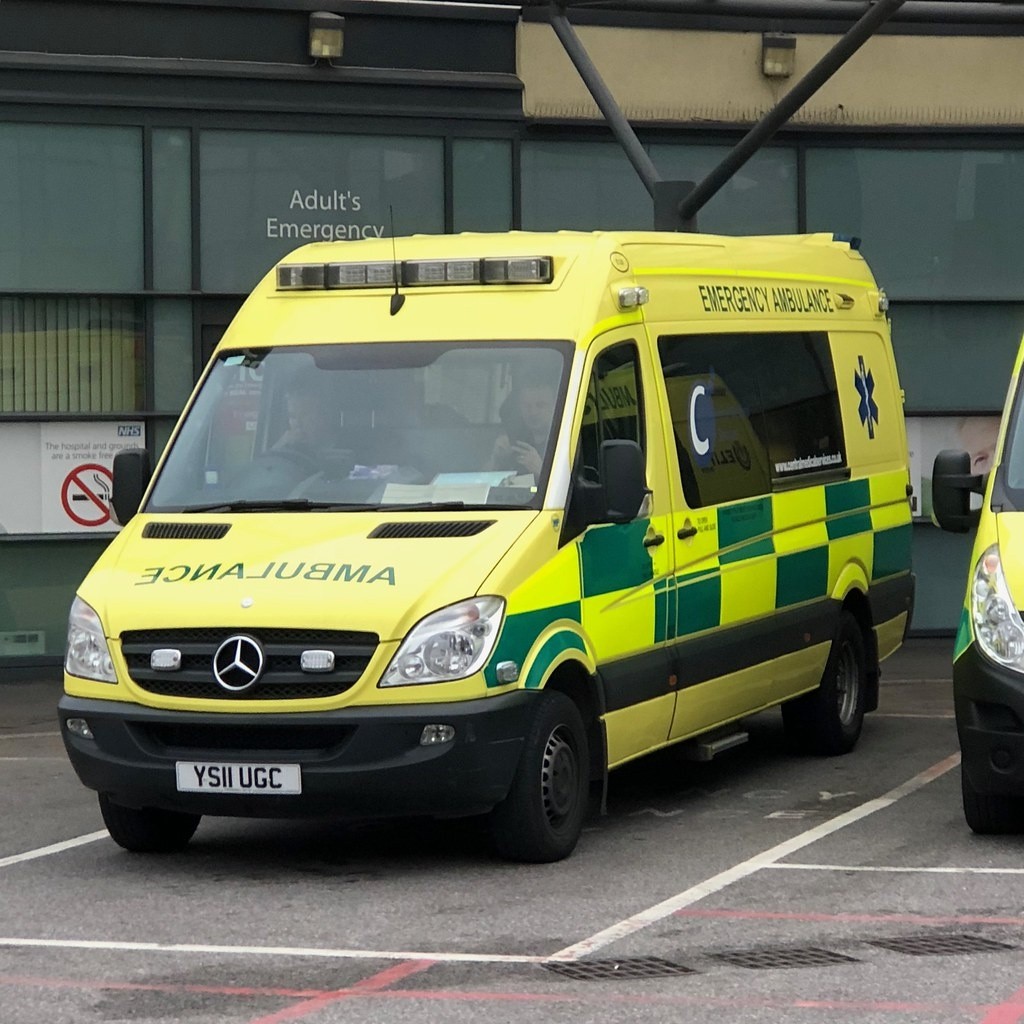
[369,367,444,470]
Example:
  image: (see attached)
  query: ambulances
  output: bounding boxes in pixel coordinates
[927,324,1022,842]
[58,226,914,868]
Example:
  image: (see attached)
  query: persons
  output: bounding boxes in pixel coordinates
[247,377,588,483]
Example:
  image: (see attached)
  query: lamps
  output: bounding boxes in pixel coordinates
[759,32,797,79]
[306,10,345,58]
